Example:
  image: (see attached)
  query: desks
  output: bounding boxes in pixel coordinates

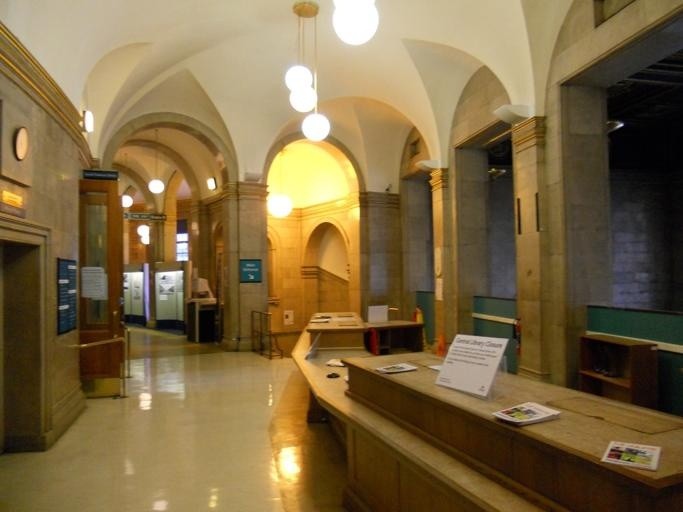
[190,297,220,343]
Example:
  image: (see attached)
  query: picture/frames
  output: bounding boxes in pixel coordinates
[240,258,262,283]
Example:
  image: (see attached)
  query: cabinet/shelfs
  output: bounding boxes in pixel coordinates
[577,334,658,410]
[76,180,126,397]
[363,320,425,355]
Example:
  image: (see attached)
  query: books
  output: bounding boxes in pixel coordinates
[309,313,359,326]
[490,400,554,423]
[326,357,346,367]
[493,401,561,427]
[600,440,663,471]
[374,361,417,374]
